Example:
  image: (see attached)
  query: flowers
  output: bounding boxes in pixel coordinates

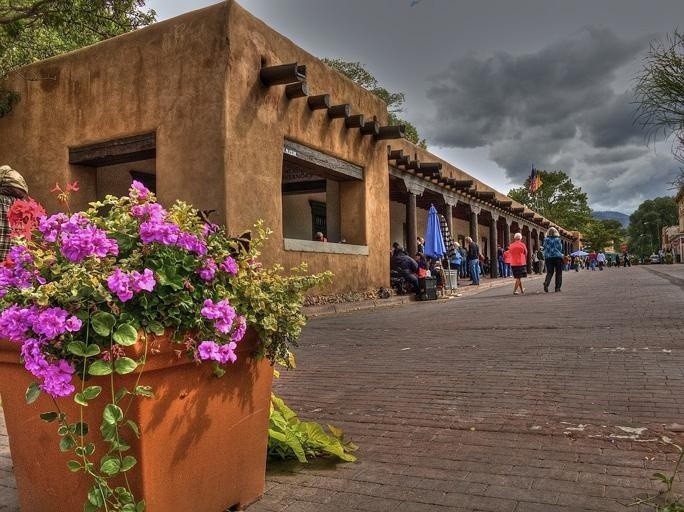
[0,176,359,509]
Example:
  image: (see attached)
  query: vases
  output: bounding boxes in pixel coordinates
[0,321,276,511]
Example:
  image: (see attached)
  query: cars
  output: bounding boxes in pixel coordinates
[649,254,659,263]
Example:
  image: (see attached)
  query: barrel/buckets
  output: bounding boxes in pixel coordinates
[445,269,459,290]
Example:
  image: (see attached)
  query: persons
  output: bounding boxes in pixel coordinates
[389,234,675,303]
[541,226,564,293]
[509,231,528,295]
[0,164,31,264]
[313,231,324,242]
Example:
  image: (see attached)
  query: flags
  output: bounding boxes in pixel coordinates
[529,162,543,194]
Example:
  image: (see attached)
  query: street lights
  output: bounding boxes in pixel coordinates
[639,234,653,253]
[643,221,661,250]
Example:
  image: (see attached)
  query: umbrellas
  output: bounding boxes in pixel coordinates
[422,203,447,296]
[438,212,462,294]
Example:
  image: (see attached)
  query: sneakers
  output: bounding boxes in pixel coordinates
[555,288,561,292]
[543,281,549,292]
[514,288,525,295]
